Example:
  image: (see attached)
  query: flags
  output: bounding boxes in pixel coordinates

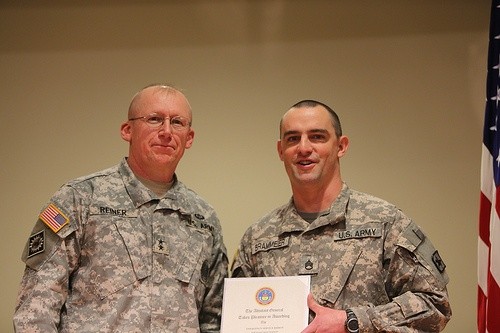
[476,1,500,332]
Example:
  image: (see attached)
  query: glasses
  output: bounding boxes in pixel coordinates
[129,114,191,132]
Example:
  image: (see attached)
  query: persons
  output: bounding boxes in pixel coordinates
[10,84,229,333]
[224,99,451,332]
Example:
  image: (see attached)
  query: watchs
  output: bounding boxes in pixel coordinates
[344,308,360,332]
